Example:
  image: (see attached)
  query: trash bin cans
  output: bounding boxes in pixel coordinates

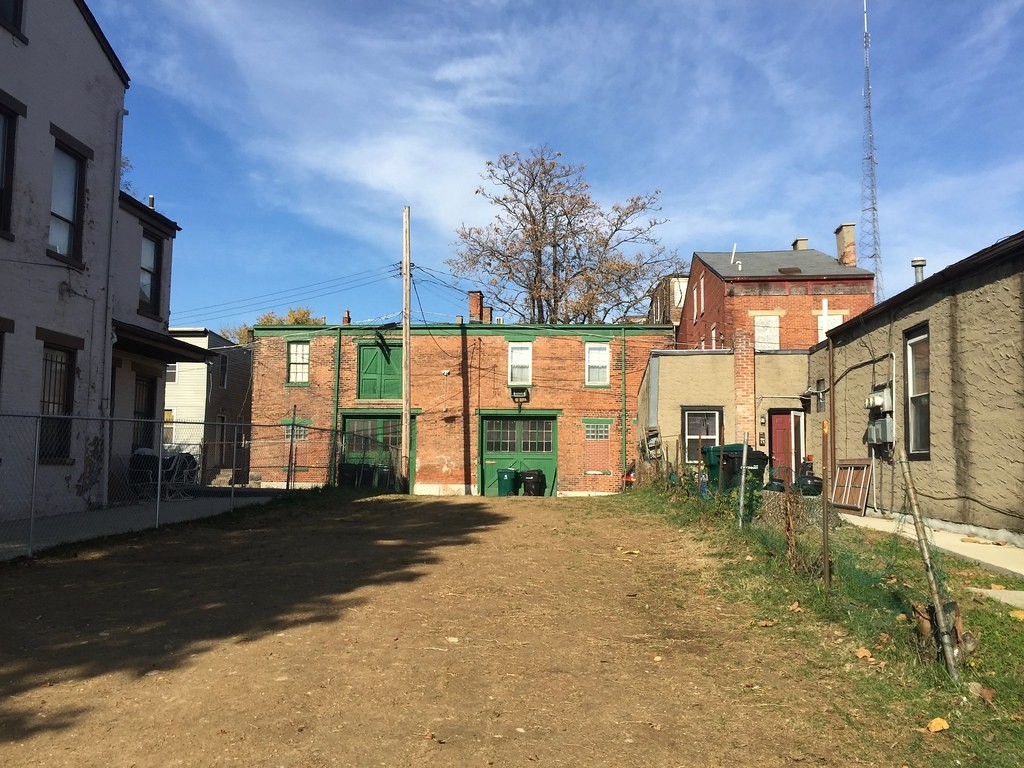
[796,474,823,496]
[340,463,395,491]
[523,469,543,496]
[716,450,768,494]
[701,444,753,493]
[497,468,516,497]
[768,478,785,492]
